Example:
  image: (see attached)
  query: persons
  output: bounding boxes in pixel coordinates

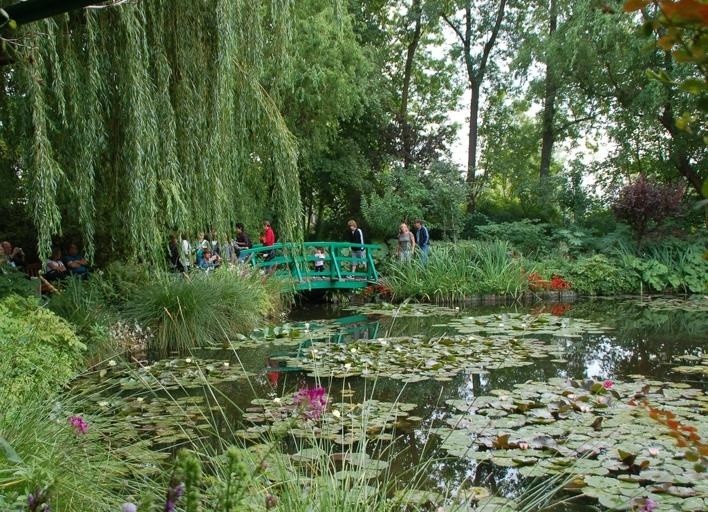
[412,219,431,268]
[258,221,276,261]
[66,243,90,275]
[347,219,368,276]
[0,245,8,277]
[394,222,416,263]
[167,229,231,274]
[232,223,252,267]
[46,246,67,281]
[311,247,325,279]
[0,240,19,276]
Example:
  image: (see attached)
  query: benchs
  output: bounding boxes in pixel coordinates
[23,263,89,298]
